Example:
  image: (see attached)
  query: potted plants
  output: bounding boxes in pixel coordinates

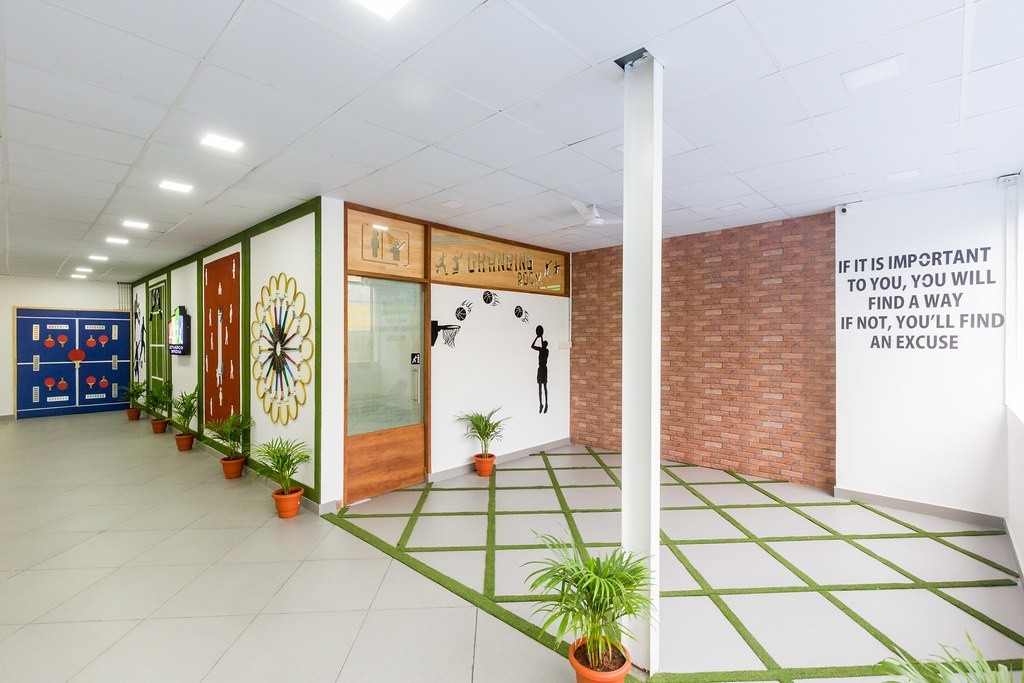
[198,414,256,479]
[454,406,513,476]
[119,380,148,420]
[519,530,659,683]
[249,437,312,518]
[144,381,174,433]
[166,384,201,451]
